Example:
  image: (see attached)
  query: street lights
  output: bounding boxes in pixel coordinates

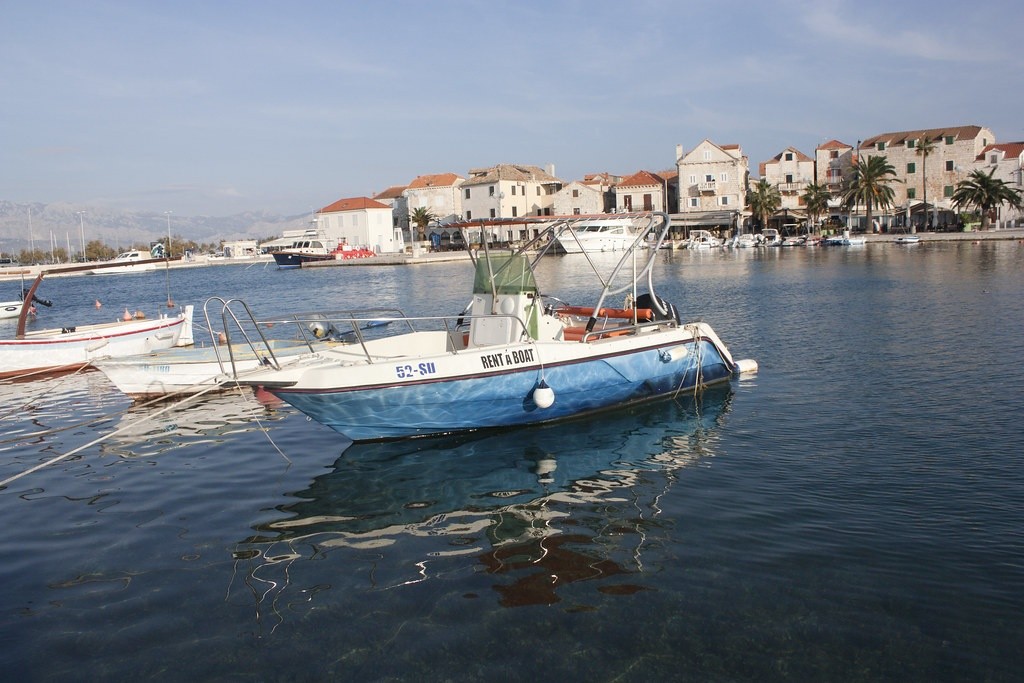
[165,209,174,258]
[77,211,89,262]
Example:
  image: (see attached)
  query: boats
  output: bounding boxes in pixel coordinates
[553,220,645,252]
[657,226,867,248]
[86,307,448,399]
[0,300,25,320]
[271,238,334,270]
[236,382,734,547]
[896,235,920,243]
[215,212,758,444]
[0,307,195,377]
